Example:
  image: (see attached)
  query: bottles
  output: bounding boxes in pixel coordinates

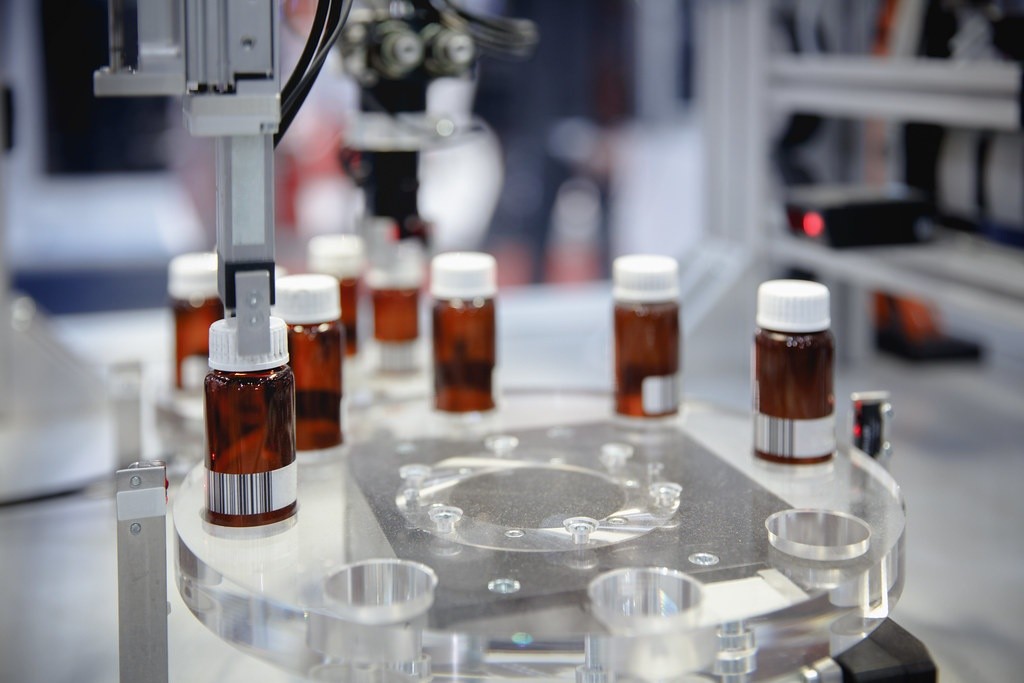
[752,279,837,466]
[163,231,425,455]
[203,314,299,528]
[612,255,680,419]
[428,251,497,413]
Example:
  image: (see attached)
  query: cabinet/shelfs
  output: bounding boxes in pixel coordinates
[677,53,1024,387]
